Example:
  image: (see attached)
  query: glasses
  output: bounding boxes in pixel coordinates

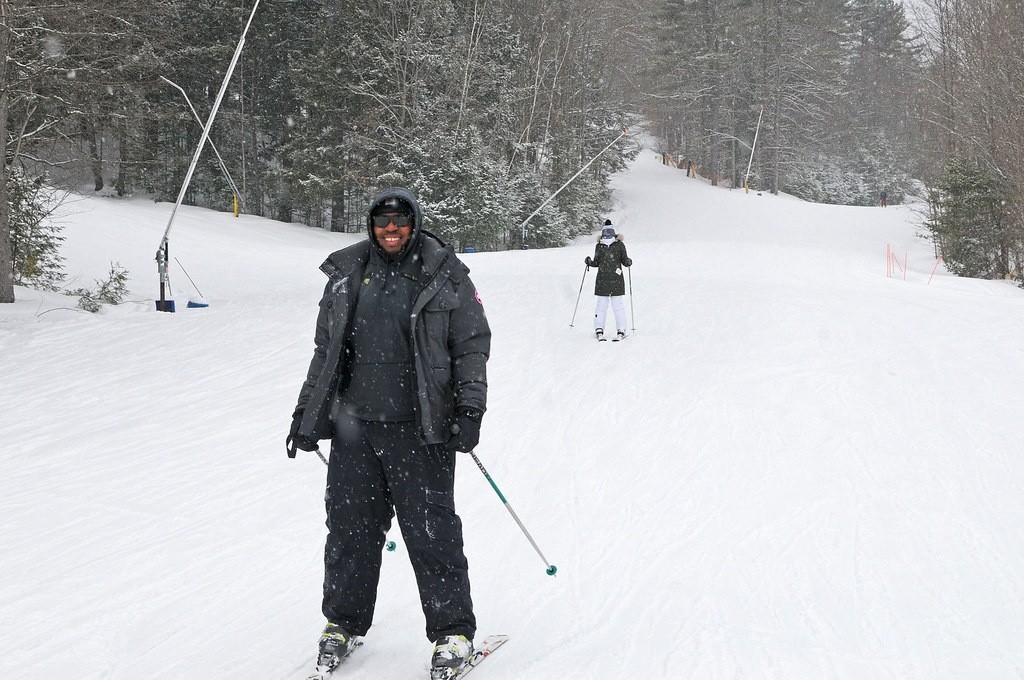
[372,215,410,228]
[603,235,613,239]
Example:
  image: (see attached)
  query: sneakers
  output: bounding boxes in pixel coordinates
[431,635,473,668]
[319,623,357,656]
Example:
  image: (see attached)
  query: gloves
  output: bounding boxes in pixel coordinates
[290,410,320,452]
[444,408,484,454]
[625,259,632,266]
[585,257,591,265]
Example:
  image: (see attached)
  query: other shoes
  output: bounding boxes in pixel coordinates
[618,329,625,339]
[596,328,603,340]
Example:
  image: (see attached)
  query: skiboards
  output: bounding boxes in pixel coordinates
[303,632,511,680]
[596,332,631,342]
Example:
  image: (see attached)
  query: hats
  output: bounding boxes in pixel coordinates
[372,198,413,217]
[596,220,623,242]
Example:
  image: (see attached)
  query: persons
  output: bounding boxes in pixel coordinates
[289,187,492,679]
[585,219,632,342]
